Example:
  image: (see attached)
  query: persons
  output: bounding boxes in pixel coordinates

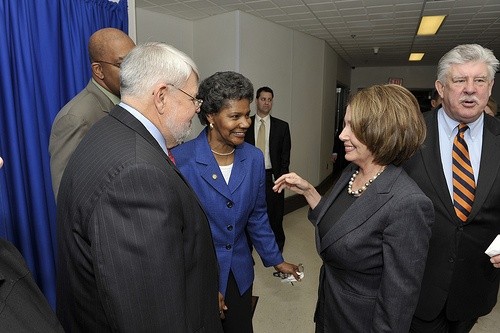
[0,156,65,333]
[272,83,435,333]
[401,43,500,333]
[330,90,497,183]
[55,41,221,333]
[167,71,302,333]
[244,86,291,266]
[49,28,138,206]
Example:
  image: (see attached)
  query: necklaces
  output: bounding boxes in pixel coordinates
[211,148,235,156]
[347,169,383,195]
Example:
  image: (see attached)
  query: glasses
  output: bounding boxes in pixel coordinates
[152,82,203,110]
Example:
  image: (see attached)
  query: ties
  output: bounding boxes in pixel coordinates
[452,123,476,222]
[167,148,175,165]
[257,119,266,154]
